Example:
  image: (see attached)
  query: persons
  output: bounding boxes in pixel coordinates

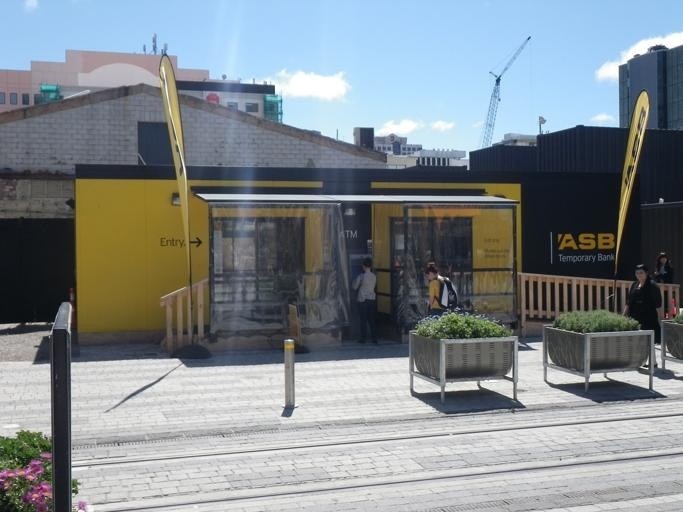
[622,264,662,369]
[425,262,449,317]
[651,253,674,319]
[351,258,378,343]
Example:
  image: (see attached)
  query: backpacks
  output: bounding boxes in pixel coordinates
[434,275,458,309]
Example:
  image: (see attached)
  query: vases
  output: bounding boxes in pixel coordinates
[408,307,519,405]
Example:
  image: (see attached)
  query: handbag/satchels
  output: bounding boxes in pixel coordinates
[622,303,630,317]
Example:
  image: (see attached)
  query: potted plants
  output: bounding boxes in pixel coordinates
[542,310,655,393]
[660,314,683,374]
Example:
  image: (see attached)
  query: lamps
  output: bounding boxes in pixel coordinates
[172,193,181,205]
[64,198,74,211]
